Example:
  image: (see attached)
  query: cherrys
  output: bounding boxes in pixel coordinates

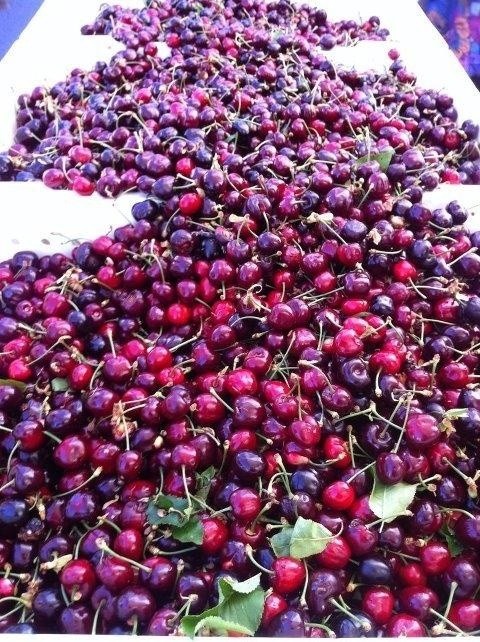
[0,0,480,637]
